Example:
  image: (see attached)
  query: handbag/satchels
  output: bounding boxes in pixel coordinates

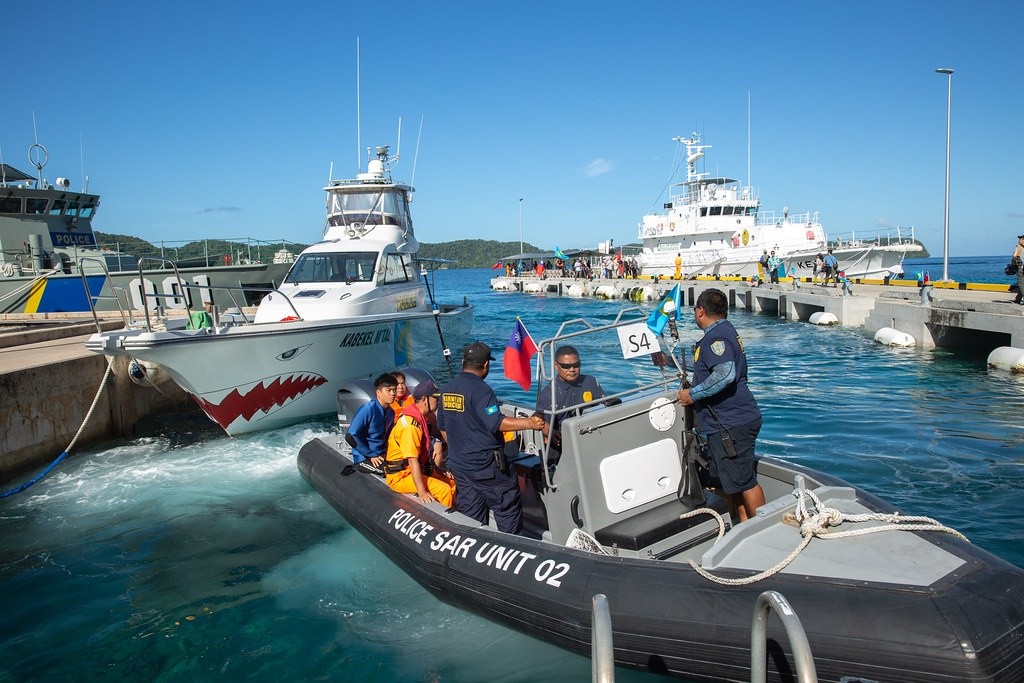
[834,263,838,268]
[813,263,816,271]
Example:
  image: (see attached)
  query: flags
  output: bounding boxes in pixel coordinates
[503,321,539,391]
[646,283,681,336]
[555,246,569,259]
[491,260,503,270]
[915,271,928,284]
[536,260,545,276]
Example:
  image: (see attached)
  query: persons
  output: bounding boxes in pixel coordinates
[675,253,682,280]
[540,346,622,464]
[344,374,399,470]
[760,250,779,284]
[812,250,837,287]
[651,288,766,523]
[544,258,566,277]
[1013,235,1024,305]
[572,259,591,280]
[507,261,537,271]
[383,379,457,508]
[390,371,413,423]
[606,257,638,279]
[436,341,546,536]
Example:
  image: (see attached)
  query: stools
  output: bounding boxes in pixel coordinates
[506,452,543,498]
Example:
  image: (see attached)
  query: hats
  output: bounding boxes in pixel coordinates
[413,379,443,400]
[816,253,822,256]
[464,342,497,364]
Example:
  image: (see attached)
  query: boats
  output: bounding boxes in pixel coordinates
[297,302,1023,682]
[624,133,927,285]
[81,144,476,437]
[0,143,332,323]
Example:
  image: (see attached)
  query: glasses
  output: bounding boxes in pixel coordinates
[555,359,582,369]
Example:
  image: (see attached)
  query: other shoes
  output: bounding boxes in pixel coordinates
[834,284,837,287]
[1012,299,1021,304]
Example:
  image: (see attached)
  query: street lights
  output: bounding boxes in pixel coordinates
[937,67,954,280]
[520,199,524,253]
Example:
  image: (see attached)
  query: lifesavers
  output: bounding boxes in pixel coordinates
[806,231,815,240]
[732,236,739,247]
[670,222,675,231]
[657,223,663,231]
